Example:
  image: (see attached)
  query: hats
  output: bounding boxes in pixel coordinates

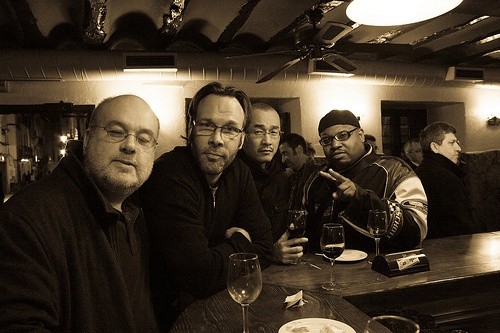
[318,109,361,137]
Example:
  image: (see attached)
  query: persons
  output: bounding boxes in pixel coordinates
[0,95,159,333]
[414,121,478,241]
[302,110,429,248]
[139,80,308,333]
[403,138,424,171]
[279,133,322,223]
[364,135,377,154]
[238,102,289,242]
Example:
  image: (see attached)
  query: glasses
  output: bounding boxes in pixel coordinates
[90,125,159,150]
[190,118,244,140]
[319,127,358,147]
[242,126,285,139]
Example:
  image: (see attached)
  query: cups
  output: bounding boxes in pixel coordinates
[364,315,419,333]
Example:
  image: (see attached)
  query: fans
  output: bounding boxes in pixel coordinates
[224,11,359,84]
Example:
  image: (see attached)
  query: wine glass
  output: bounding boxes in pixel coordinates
[226,253,262,333]
[286,210,306,264]
[320,223,345,290]
[368,210,389,263]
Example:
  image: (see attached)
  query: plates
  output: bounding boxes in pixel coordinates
[324,248,368,262]
[278,318,355,333]
